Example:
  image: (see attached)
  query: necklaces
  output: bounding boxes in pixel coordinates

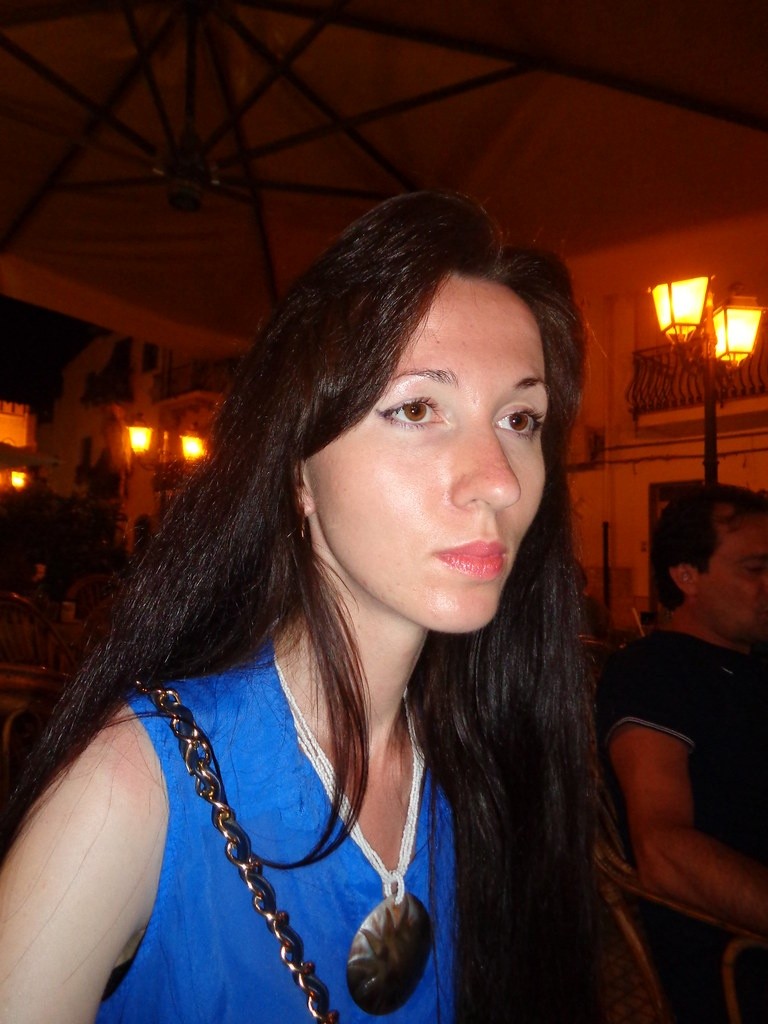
[272,649,437,1017]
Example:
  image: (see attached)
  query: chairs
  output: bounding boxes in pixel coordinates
[0,574,120,797]
[576,634,768,1024]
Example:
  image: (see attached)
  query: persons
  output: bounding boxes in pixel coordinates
[0,185,615,1023]
[600,476,767,1024]
